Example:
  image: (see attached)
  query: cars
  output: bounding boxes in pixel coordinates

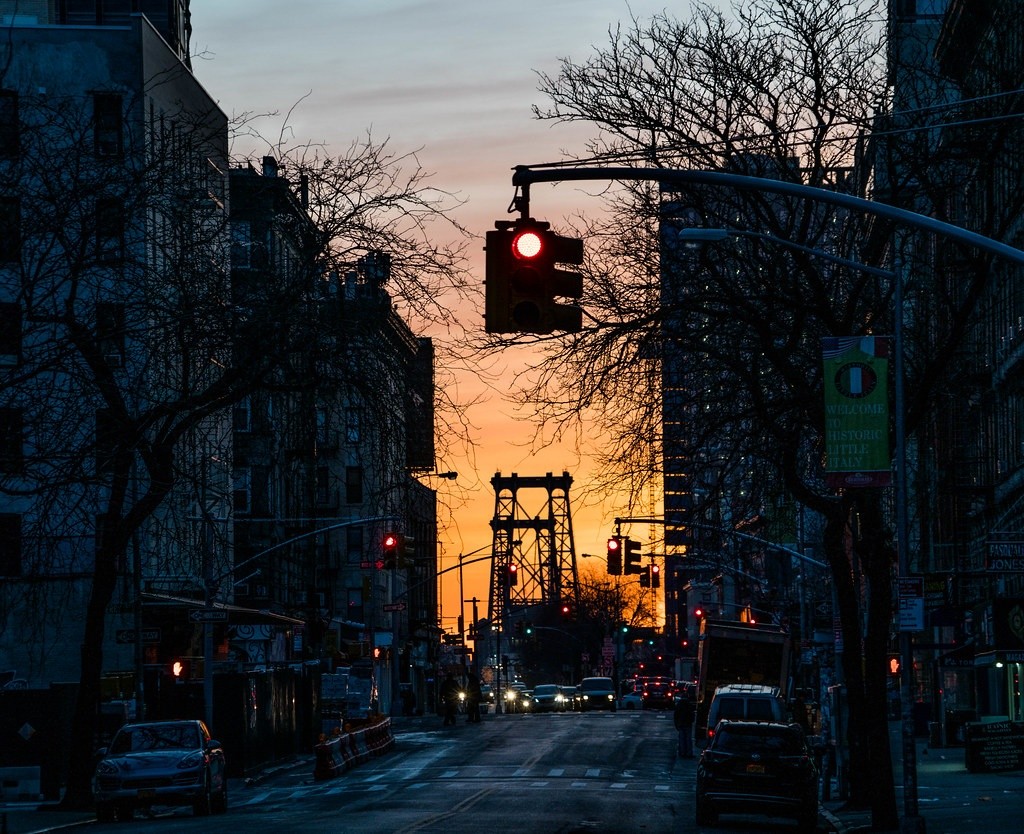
[581,677,617,712]
[479,682,511,715]
[500,683,589,714]
[91,719,228,824]
[620,674,695,710]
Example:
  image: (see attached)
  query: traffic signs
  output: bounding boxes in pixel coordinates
[186,607,230,626]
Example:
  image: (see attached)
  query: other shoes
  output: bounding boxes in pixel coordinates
[683,754,696,758]
[473,720,480,723]
[465,719,471,722]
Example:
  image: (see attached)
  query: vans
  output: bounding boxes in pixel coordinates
[707,682,789,750]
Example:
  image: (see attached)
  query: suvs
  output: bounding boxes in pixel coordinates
[695,719,818,827]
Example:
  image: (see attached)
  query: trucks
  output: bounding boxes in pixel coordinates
[673,618,798,739]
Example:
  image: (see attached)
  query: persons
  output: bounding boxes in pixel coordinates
[132,729,194,750]
[438,670,462,726]
[674,693,695,756]
[464,664,482,723]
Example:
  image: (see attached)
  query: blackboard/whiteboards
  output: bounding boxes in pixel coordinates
[964,720,1024,773]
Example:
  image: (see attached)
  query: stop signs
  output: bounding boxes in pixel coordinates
[821,355,890,473]
[883,653,907,678]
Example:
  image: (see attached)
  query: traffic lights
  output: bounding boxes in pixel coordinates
[638,565,649,587]
[693,606,703,620]
[380,531,398,570]
[394,528,415,569]
[747,619,757,627]
[496,565,508,586]
[542,227,583,335]
[507,563,519,586]
[560,605,571,616]
[651,563,660,587]
[618,625,630,636]
[624,539,642,574]
[370,646,383,664]
[496,229,555,332]
[522,624,536,637]
[606,538,622,575]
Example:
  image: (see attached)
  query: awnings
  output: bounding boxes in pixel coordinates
[924,644,972,671]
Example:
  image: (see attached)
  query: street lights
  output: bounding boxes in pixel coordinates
[361,472,459,718]
[679,227,929,833]
